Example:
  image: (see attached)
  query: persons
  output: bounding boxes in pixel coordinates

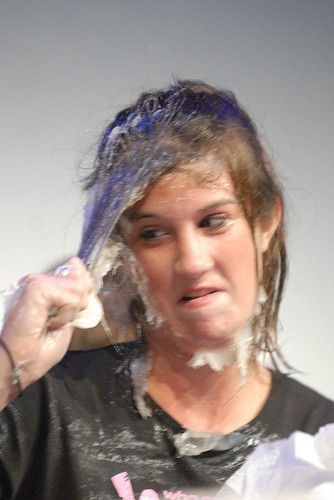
[0,78,334,500]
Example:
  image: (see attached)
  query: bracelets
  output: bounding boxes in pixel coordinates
[0,338,23,397]
